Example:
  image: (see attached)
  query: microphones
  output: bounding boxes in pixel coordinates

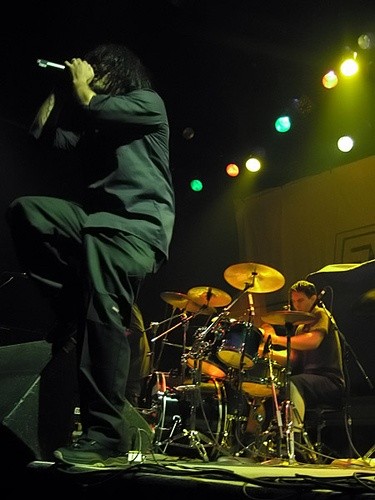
[37,58,67,71]
[309,288,325,313]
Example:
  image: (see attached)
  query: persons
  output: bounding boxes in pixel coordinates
[247,280,347,456]
[7,41,176,471]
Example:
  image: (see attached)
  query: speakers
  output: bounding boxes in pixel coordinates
[0,341,54,459]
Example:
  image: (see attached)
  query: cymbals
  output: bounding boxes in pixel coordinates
[159,292,217,316]
[187,286,232,308]
[223,262,285,294]
[261,310,317,326]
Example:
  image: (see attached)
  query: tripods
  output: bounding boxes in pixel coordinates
[154,286,318,466]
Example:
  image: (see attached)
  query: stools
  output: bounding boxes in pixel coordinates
[307,406,354,461]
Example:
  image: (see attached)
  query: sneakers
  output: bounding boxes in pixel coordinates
[52,434,138,471]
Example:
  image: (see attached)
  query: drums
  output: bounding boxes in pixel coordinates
[225,413,248,448]
[143,371,228,461]
[238,356,286,398]
[215,321,264,369]
[186,315,237,378]
